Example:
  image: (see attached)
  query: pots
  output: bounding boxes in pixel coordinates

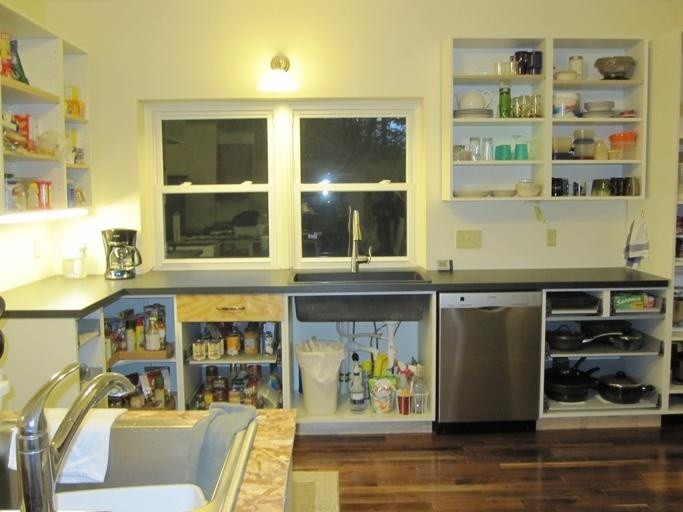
[611,328,643,351]
[577,321,631,345]
[547,367,600,403]
[592,371,655,405]
[543,357,589,392]
[552,324,623,351]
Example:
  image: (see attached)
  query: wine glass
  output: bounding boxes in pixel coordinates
[510,135,521,159]
[526,140,533,160]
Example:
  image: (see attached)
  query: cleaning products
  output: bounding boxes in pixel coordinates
[408,365,417,391]
[396,360,411,413]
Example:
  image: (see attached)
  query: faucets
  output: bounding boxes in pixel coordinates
[17,364,136,511]
[352,210,373,273]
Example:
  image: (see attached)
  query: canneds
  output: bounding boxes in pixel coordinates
[196,363,260,410]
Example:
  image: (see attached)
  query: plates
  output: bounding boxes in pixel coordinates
[489,191,517,197]
[454,109,494,118]
[583,110,613,118]
[585,101,616,112]
[453,190,490,197]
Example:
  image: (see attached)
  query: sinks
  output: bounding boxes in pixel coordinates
[289,267,431,322]
[0,418,257,511]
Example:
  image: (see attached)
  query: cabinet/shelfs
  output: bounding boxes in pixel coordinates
[283,291,437,436]
[537,287,669,430]
[0,308,104,409]
[0,0,93,218]
[668,135,683,415]
[102,294,183,410]
[177,295,288,411]
[440,35,650,202]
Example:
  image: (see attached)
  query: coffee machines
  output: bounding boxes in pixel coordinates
[101,228,142,280]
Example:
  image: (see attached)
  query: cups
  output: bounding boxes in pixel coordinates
[610,177,625,194]
[515,144,527,160]
[398,396,412,415]
[495,146,500,159]
[509,60,517,74]
[499,145,512,160]
[624,177,640,196]
[591,179,596,195]
[469,137,480,160]
[553,135,572,152]
[494,62,505,74]
[482,138,493,162]
[596,179,613,196]
[453,144,465,160]
[573,176,586,197]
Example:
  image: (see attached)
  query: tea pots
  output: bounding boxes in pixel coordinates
[454,88,494,109]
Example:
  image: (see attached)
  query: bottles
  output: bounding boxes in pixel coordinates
[104,311,159,364]
[154,376,164,407]
[349,374,366,410]
[499,88,511,118]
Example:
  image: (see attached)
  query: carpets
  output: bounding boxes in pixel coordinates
[287,469,340,511]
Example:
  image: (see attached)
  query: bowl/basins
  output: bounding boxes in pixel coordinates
[556,71,578,79]
[516,184,541,197]
[554,92,579,113]
[596,56,634,80]
